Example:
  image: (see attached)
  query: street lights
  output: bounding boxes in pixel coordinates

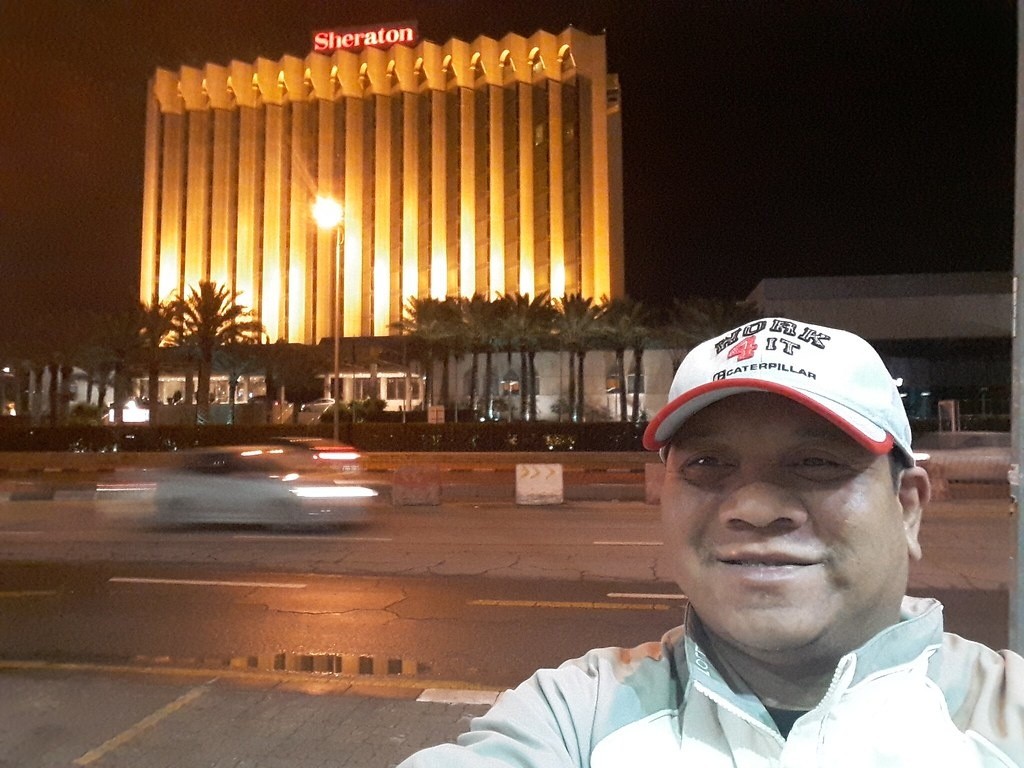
[310,190,346,441]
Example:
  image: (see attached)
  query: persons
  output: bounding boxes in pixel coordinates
[396,315,1024,768]
[167,391,185,405]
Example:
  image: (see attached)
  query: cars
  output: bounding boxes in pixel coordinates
[150,443,382,536]
[300,397,336,412]
[264,435,373,477]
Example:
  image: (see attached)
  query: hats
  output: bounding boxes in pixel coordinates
[643,317,914,469]
